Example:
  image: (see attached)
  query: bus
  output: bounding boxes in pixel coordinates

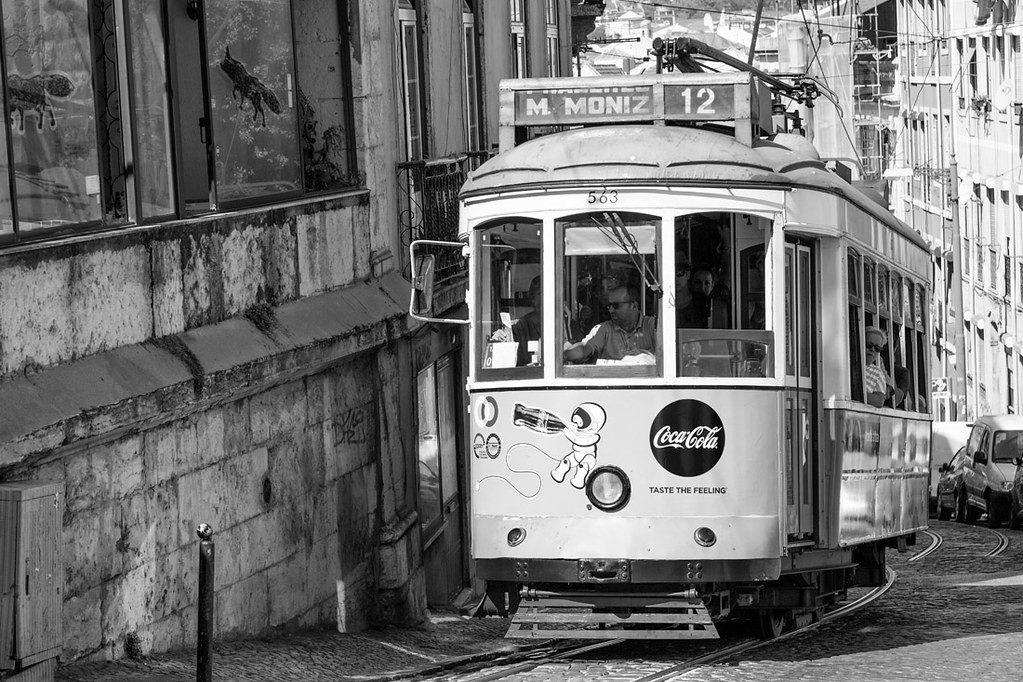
[397,0,953,645]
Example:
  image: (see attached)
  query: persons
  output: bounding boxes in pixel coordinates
[864,325,887,407]
[563,284,657,363]
[877,357,896,401]
[675,253,764,377]
[574,257,610,337]
[893,354,910,409]
[490,275,582,368]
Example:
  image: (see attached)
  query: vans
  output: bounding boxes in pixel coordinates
[961,413,1023,528]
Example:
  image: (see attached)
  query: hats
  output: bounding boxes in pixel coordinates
[601,269,625,283]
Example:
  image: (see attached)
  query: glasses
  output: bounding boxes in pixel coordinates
[866,342,883,353]
[606,300,633,309]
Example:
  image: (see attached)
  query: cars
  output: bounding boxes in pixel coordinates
[936,446,967,522]
